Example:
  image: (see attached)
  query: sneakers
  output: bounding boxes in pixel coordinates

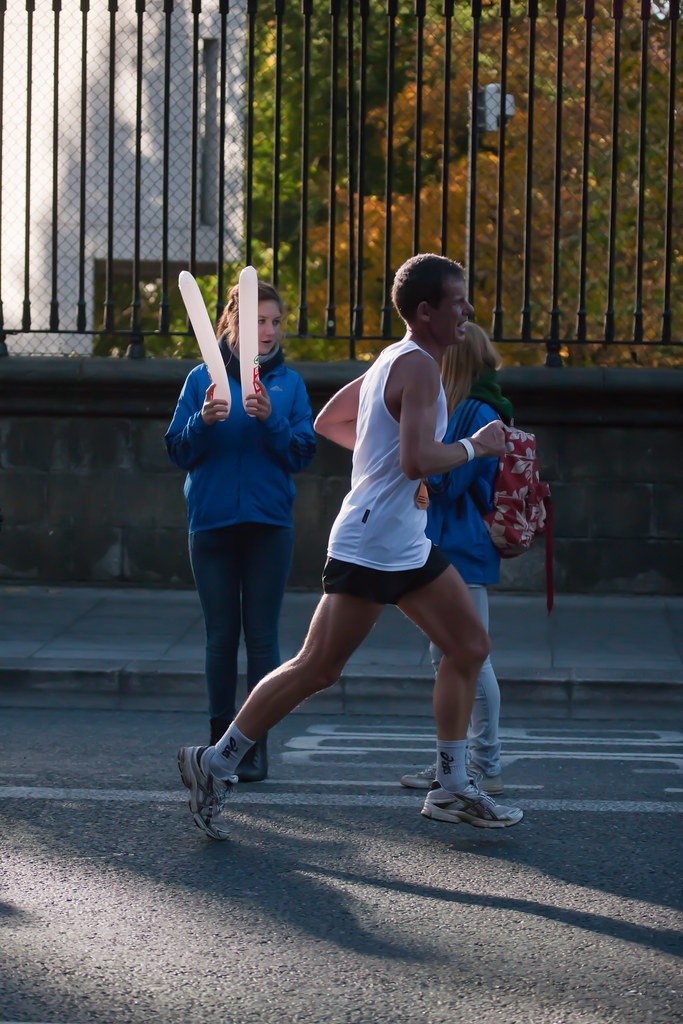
[420,780,524,829]
[400,764,440,789]
[465,761,505,795]
[178,745,239,841]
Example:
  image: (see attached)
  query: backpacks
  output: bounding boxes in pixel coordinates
[468,420,557,616]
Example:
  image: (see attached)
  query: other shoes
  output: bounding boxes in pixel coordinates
[234,738,269,782]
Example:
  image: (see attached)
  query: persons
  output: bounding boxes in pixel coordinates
[164,281,318,784]
[400,320,504,799]
[173,251,524,842]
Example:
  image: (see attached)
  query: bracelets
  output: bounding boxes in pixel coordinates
[458,438,475,462]
